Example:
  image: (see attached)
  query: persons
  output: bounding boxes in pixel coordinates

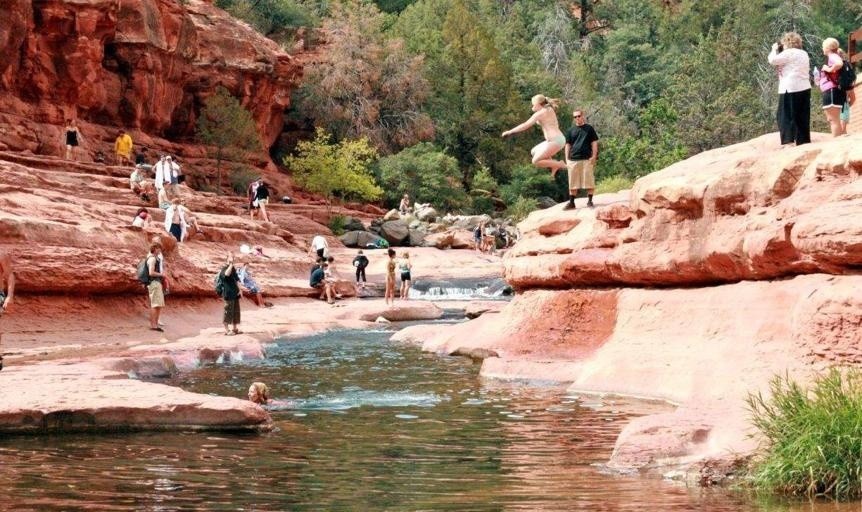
[114,129,133,169]
[836,48,850,135]
[255,182,270,224]
[0,255,17,370]
[470,219,517,256]
[352,249,370,288]
[247,180,260,222]
[247,381,290,409]
[501,94,571,178]
[812,37,845,137]
[219,251,246,337]
[65,119,84,161]
[398,193,413,214]
[767,30,813,146]
[384,248,397,306]
[398,251,413,301]
[129,148,207,333]
[239,262,266,308]
[563,108,599,212]
[307,233,344,305]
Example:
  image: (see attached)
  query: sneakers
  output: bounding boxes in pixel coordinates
[563,202,575,210]
[587,200,593,207]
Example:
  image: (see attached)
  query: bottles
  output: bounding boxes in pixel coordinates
[813,66,820,87]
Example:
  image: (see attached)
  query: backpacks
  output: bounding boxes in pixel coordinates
[136,255,160,284]
[824,59,856,113]
[213,263,229,297]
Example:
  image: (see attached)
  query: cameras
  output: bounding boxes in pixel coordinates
[778,41,782,52]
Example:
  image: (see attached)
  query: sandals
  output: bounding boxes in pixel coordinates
[224,330,236,336]
[232,329,244,334]
